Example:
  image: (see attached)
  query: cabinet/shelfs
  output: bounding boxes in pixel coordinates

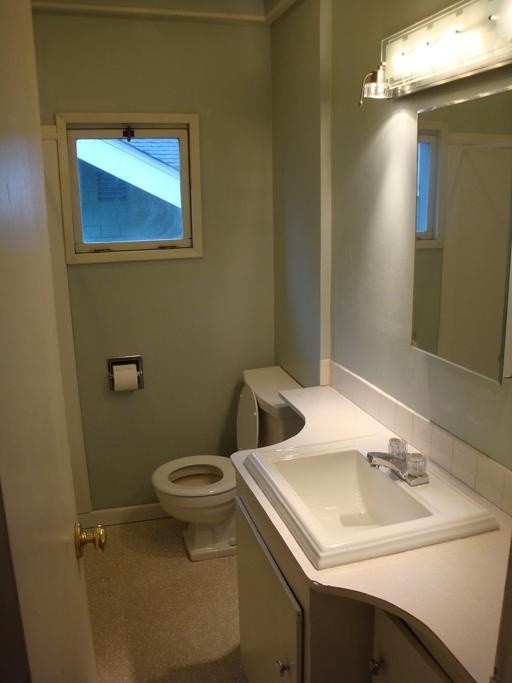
[232,469,374,681]
[368,606,454,682]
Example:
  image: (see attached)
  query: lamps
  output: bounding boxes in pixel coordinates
[353,0,512,106]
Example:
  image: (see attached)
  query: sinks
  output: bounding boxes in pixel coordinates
[250,442,439,554]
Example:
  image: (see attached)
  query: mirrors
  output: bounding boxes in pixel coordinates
[406,84,512,386]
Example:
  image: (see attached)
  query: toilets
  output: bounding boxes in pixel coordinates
[151,363,305,563]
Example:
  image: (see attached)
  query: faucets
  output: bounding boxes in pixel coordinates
[366,436,431,486]
